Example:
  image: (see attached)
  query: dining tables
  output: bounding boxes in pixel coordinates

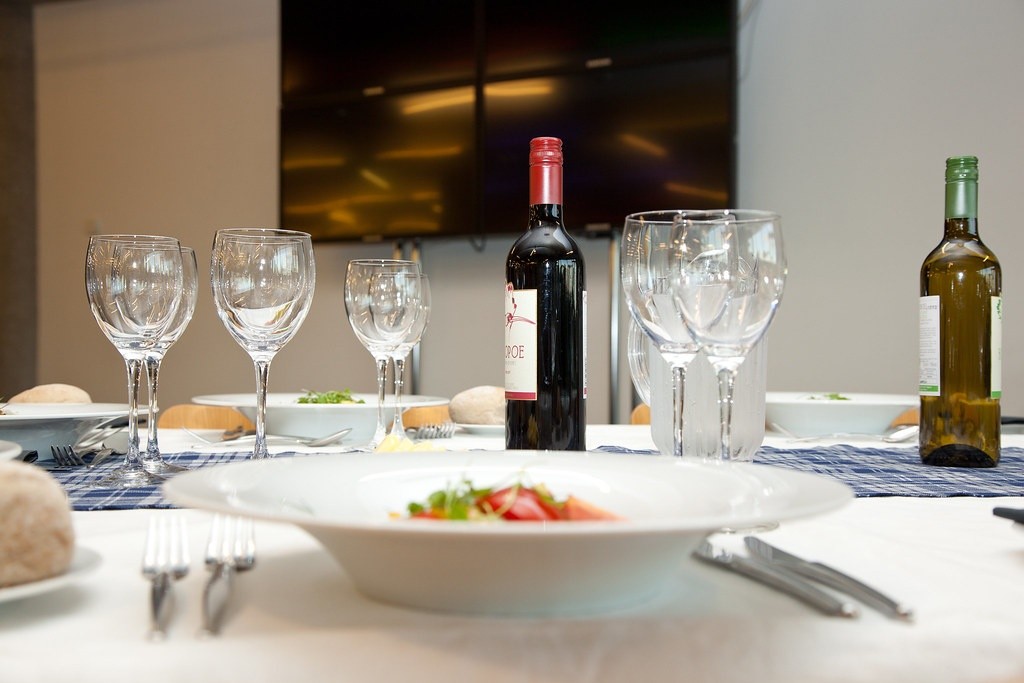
[0,422,1024,683]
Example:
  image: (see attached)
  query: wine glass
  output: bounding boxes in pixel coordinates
[623,209,786,462]
[87,233,200,489]
[210,229,316,460]
[343,259,432,452]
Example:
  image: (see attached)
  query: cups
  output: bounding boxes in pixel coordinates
[648,333,765,463]
[100,429,140,454]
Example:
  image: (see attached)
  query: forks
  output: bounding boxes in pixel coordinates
[203,511,257,632]
[50,445,103,466]
[404,423,456,439]
[143,511,189,636]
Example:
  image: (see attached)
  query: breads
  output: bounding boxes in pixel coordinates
[0,457,76,589]
[9,383,92,404]
[450,385,507,425]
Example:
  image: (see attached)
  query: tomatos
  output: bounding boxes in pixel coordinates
[484,488,564,520]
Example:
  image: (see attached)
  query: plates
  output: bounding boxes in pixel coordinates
[455,421,507,436]
[164,451,856,618]
[0,544,105,602]
[763,393,920,439]
[0,401,159,461]
[191,391,449,445]
[0,438,22,462]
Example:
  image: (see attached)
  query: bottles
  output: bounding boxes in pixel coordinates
[503,137,586,451]
[918,155,1004,468]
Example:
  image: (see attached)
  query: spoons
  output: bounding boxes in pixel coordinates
[219,427,243,442]
[249,429,353,447]
[831,426,919,442]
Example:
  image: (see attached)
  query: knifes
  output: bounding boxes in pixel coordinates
[744,535,912,618]
[694,540,861,620]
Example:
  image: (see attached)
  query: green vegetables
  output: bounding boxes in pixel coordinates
[297,389,367,403]
[407,480,560,520]
